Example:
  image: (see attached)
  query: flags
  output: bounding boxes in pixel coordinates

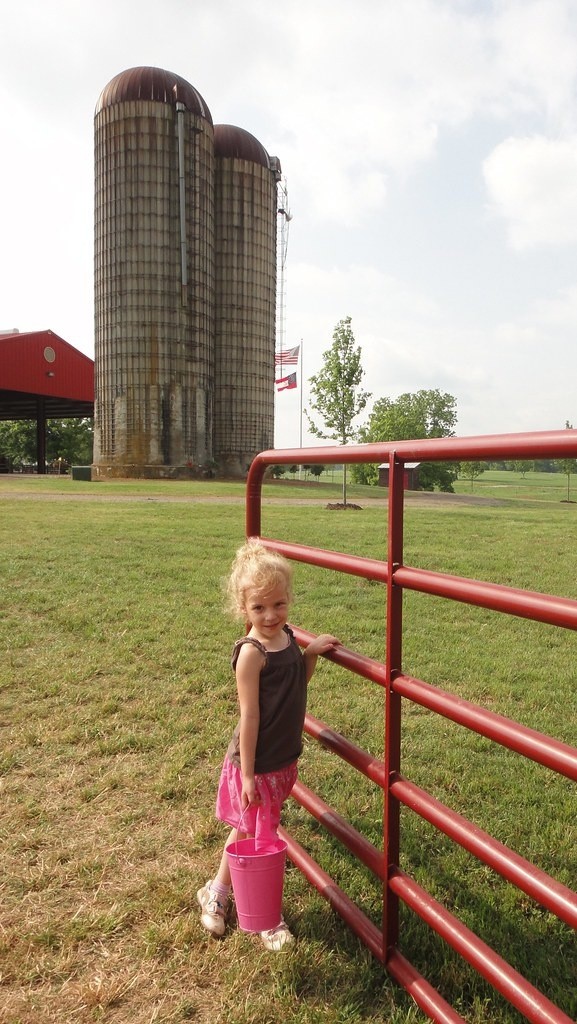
[276,372,297,392]
[274,345,299,365]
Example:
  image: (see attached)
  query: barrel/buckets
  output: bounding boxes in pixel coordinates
[226,801,287,933]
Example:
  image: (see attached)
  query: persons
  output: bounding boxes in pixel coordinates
[196,533,341,954]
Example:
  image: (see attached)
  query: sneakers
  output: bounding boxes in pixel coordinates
[197,880,231,936]
[261,913,296,951]
